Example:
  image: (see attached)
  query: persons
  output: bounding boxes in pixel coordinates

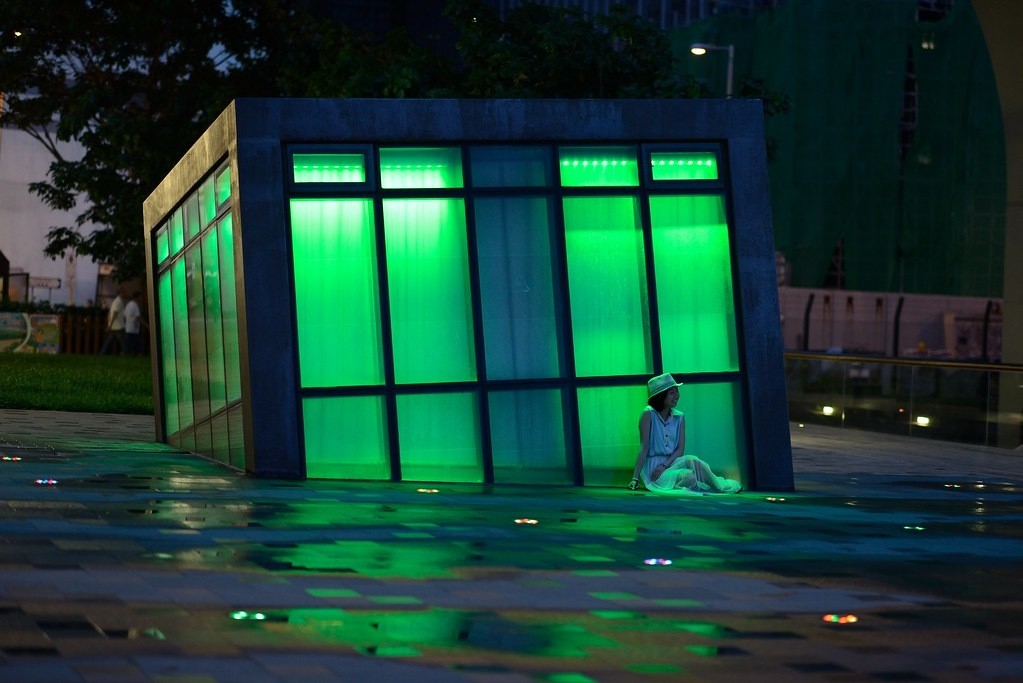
[627,373,743,494]
[98,287,149,357]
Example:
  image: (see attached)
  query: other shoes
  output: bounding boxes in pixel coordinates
[735,482,743,493]
[697,482,711,492]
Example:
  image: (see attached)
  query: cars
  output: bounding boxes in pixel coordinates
[787,348,1001,437]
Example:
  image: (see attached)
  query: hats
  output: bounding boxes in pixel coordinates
[647,373,683,401]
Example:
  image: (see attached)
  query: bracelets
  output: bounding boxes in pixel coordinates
[631,478,640,484]
[662,462,668,469]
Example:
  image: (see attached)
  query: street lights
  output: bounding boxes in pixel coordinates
[691,42,735,98]
